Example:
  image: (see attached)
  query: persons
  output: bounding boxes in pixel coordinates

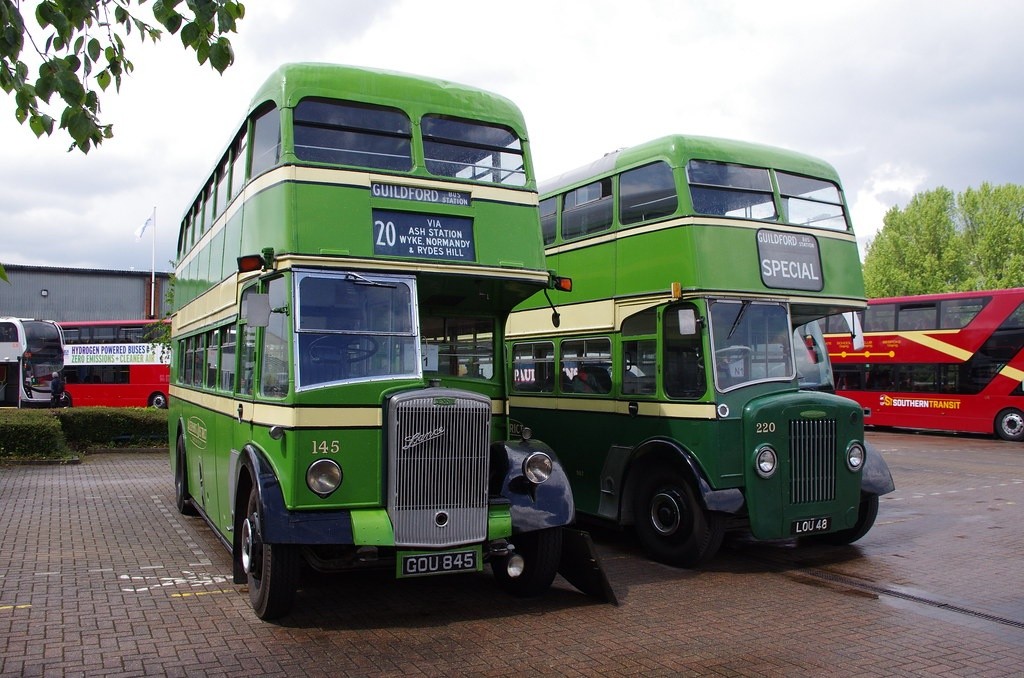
[50,371,64,408]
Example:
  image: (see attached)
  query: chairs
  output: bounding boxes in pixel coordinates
[516,382,572,393]
[65,339,129,344]
[820,318,960,334]
[178,366,278,397]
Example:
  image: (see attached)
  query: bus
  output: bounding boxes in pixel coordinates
[168,55,584,628]
[794,281,1024,443]
[0,314,66,409]
[438,136,901,573]
[49,318,179,413]
